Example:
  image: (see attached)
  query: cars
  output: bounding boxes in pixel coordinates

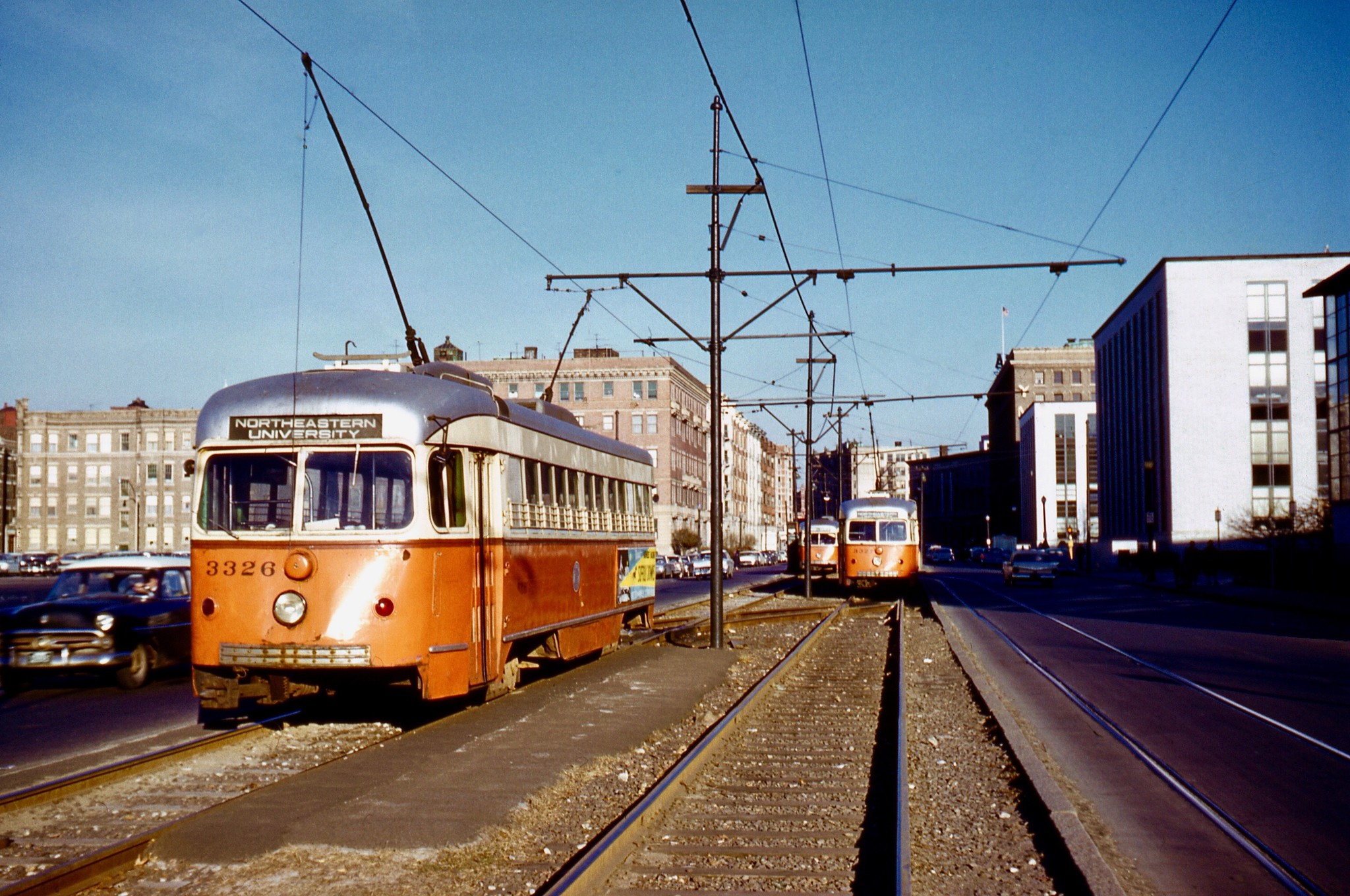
[924,542,1007,568]
[1002,549,1060,588]
[0,555,195,694]
[1044,547,1078,577]
[0,542,190,578]
[655,547,790,581]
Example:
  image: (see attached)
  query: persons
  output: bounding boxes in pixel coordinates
[132,569,167,600]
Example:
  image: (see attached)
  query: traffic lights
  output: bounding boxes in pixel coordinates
[1215,509,1222,542]
[1067,527,1072,534]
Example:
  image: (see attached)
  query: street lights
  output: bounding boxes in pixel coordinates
[919,472,927,568]
[823,473,832,516]
[984,514,991,550]
[1039,496,1050,550]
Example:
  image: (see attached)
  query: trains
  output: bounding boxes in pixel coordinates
[190,362,656,727]
[838,498,920,591]
[796,519,842,576]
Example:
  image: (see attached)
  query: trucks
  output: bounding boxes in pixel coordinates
[991,534,1017,553]
